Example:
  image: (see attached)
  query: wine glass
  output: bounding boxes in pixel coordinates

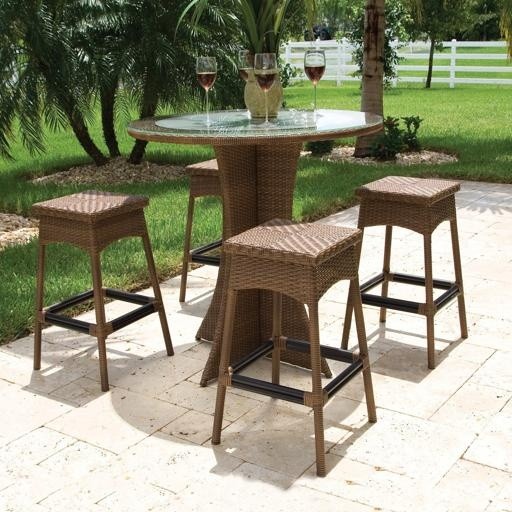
[237,48,252,116]
[195,56,218,124]
[252,54,278,128]
[304,48,326,121]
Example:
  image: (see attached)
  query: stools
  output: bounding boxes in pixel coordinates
[340,175,467,368]
[179,157,219,302]
[208,217,377,476]
[30,189,174,391]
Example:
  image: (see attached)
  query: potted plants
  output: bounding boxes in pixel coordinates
[175,0,320,120]
